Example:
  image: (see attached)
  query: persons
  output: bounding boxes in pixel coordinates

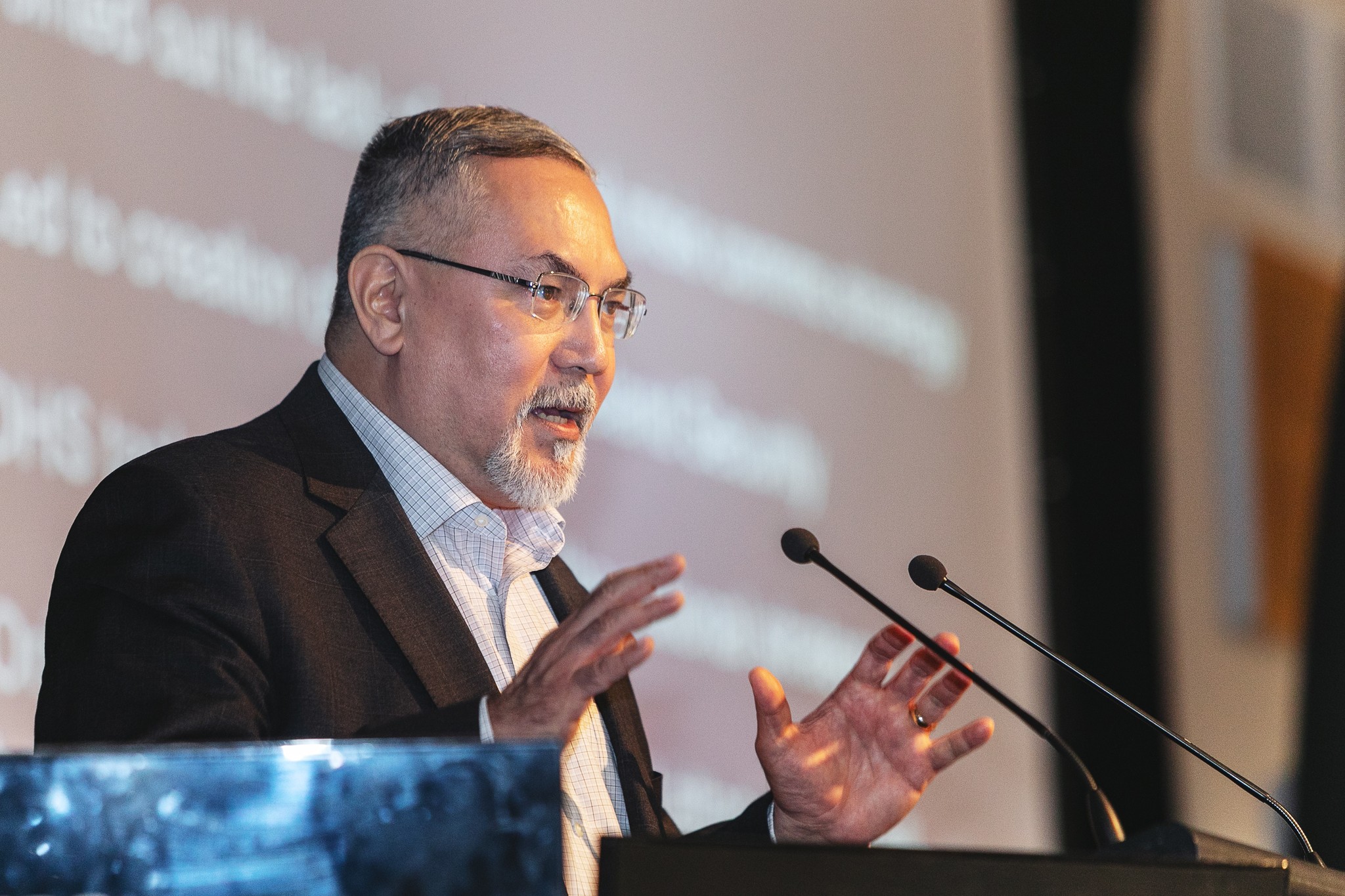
[31,101,995,895]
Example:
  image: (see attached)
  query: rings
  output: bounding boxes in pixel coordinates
[910,703,936,732]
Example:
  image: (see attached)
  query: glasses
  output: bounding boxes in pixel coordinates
[397,250,647,343]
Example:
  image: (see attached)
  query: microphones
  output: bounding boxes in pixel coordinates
[781,528,1282,865]
[902,557,1326,870]
